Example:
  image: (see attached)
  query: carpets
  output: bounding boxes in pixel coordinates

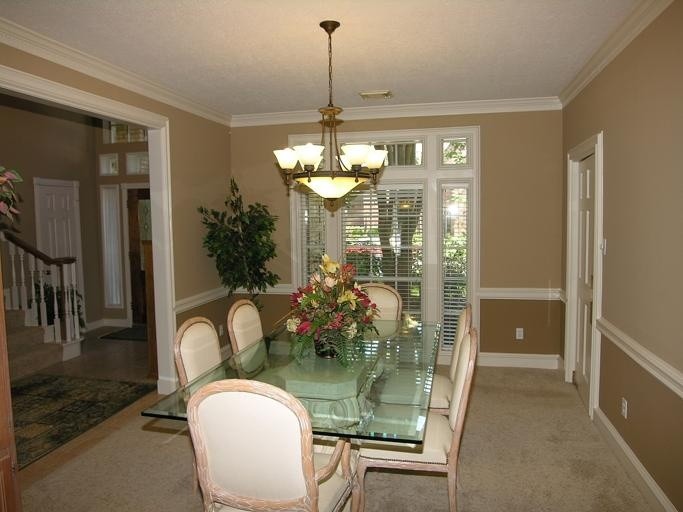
[10,371,156,471]
[100,325,147,341]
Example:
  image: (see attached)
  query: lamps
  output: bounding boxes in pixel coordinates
[271,20,390,209]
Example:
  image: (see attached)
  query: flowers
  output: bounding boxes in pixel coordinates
[0,166,23,225]
[283,251,383,370]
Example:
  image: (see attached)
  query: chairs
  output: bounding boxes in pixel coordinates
[357,283,403,371]
[375,302,475,417]
[185,378,363,512]
[172,316,226,496]
[357,329,479,511]
[227,299,287,394]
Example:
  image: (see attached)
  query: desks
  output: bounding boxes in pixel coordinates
[140,317,443,497]
